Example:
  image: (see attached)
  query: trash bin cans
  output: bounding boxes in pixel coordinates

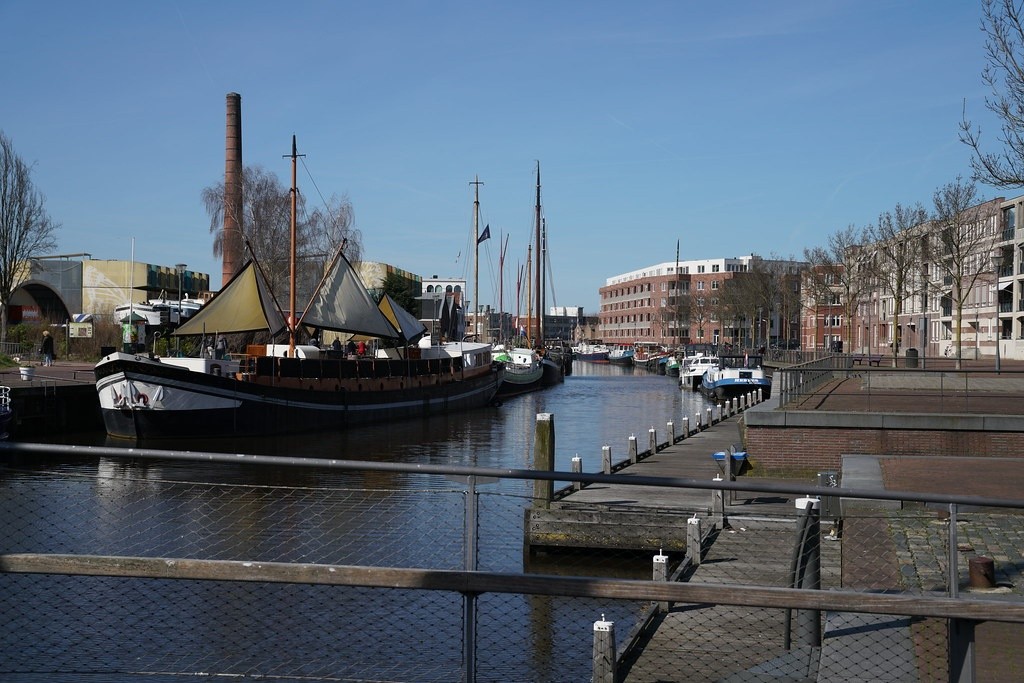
[832,341,843,352]
[905,349,919,369]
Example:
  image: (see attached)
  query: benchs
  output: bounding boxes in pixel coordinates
[852,354,884,367]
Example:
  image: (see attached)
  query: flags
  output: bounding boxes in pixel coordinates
[518,320,528,338]
[477,224,490,244]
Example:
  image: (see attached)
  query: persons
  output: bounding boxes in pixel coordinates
[332,336,341,350]
[346,340,369,355]
[309,339,320,349]
[205,335,227,360]
[39,330,54,367]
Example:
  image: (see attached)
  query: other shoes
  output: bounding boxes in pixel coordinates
[49,364,52,367]
[44,364,48,367]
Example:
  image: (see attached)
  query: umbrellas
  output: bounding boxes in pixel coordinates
[495,353,514,362]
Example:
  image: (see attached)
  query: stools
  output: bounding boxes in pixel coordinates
[713,451,746,476]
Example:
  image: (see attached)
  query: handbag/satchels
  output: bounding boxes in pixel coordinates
[51,353,57,360]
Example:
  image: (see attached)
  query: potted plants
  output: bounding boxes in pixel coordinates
[19,365,35,381]
